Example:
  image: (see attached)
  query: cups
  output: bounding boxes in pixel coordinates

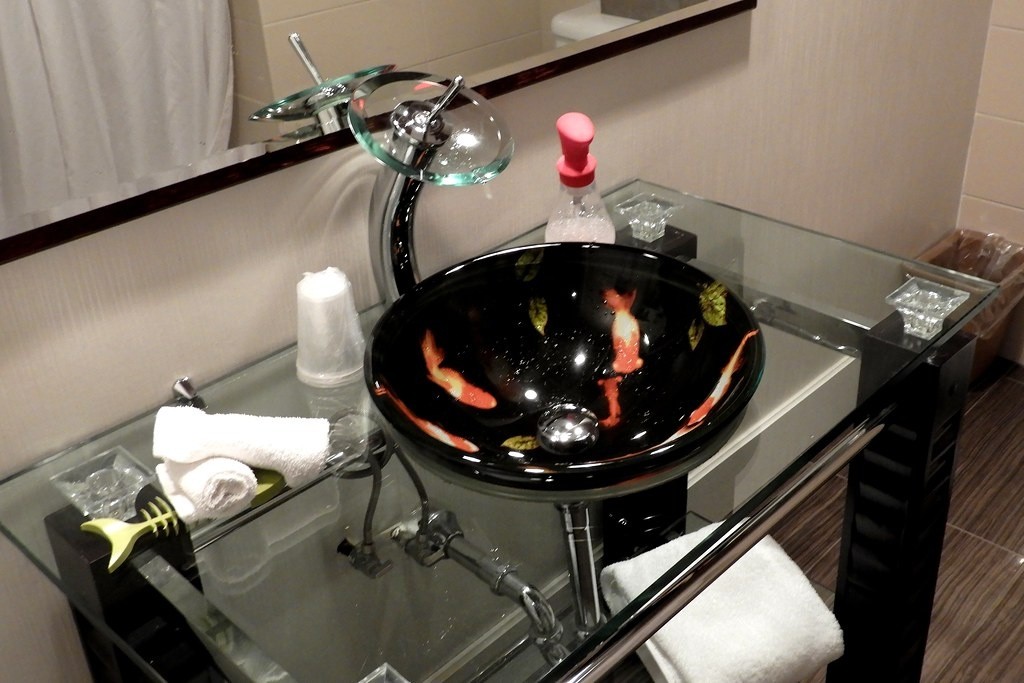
[292,270,364,386]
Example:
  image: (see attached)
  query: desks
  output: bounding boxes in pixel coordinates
[0,176,1001,683]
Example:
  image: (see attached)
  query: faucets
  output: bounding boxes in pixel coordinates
[344,68,515,304]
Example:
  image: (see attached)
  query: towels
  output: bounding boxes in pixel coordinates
[598,519,845,683]
[153,404,330,526]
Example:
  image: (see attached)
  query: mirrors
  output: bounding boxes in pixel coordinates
[0,0,757,269]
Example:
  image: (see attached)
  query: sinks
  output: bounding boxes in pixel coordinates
[363,242,768,506]
